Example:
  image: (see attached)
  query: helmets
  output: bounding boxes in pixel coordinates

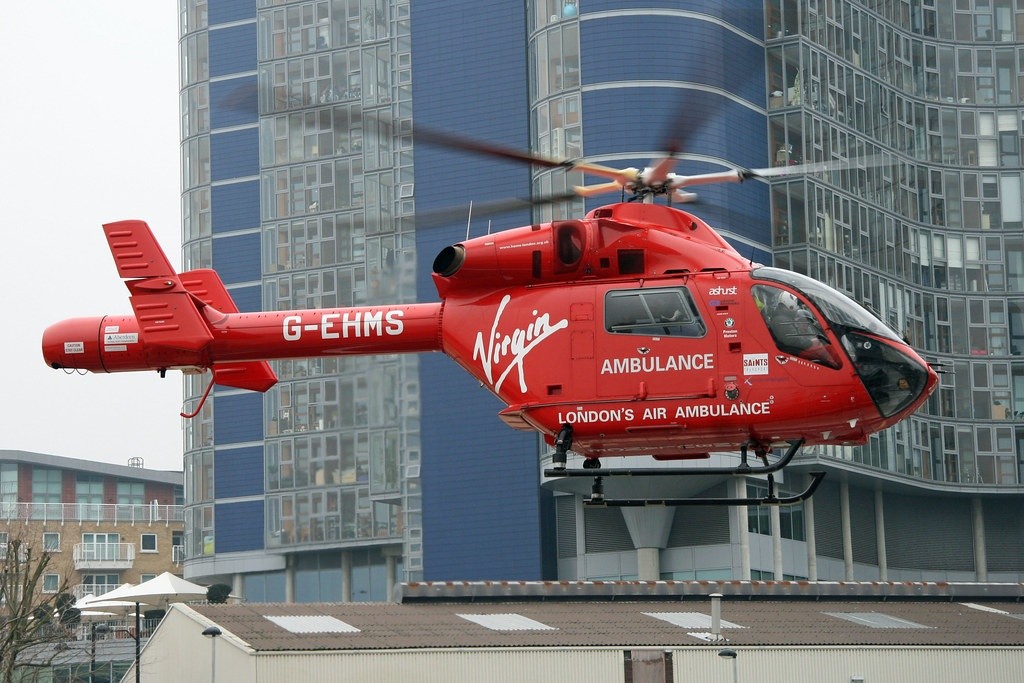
[778,290,799,312]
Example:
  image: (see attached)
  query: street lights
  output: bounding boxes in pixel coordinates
[202,628,222,683]
[94,624,141,683]
[54,643,94,683]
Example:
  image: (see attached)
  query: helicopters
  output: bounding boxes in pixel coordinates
[41,0,1024,508]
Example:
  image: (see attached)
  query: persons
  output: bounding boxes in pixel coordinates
[759,295,763,300]
[770,292,889,403]
[907,320,913,345]
[933,204,943,225]
[662,295,698,322]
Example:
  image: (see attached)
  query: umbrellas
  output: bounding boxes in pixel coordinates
[26,570,245,639]
